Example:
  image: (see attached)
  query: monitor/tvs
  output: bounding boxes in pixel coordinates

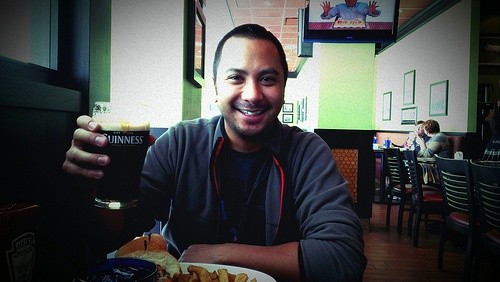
[303,0,400,43]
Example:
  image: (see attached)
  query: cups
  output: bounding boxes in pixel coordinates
[91,101,150,209]
[454,151,463,159]
[385,140,391,148]
[78,257,156,282]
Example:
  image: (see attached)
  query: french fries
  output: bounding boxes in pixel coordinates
[186,265,249,282]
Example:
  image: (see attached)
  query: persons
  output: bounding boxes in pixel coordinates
[321,0,382,21]
[62,23,368,282]
[388,119,450,199]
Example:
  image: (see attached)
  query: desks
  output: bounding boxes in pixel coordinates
[374,151,419,204]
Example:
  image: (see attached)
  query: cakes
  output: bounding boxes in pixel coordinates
[332,18,367,29]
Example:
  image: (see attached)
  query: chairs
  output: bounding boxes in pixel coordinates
[469,159,500,282]
[383,148,440,236]
[435,157,480,282]
[404,151,449,248]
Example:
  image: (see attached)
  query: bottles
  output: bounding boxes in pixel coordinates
[0,198,52,282]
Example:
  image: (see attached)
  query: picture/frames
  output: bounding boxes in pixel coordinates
[186,0,206,87]
[283,114,293,123]
[382,91,392,121]
[283,103,293,112]
[401,106,418,125]
[403,69,416,105]
[429,80,449,117]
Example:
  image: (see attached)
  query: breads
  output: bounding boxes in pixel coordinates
[114,233,182,282]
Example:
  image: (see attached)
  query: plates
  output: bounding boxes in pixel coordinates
[179,261,277,282]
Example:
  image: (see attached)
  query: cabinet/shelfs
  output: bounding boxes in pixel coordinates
[479,37,500,69]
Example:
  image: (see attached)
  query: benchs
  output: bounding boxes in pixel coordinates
[376,130,465,186]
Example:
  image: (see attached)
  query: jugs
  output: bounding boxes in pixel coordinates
[479,125,490,143]
[480,104,494,124]
[480,82,494,102]
